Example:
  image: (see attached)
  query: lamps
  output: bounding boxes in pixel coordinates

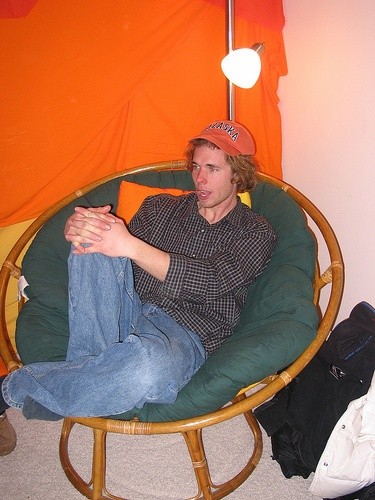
[220,42,265,90]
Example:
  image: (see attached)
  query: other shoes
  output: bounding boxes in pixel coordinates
[0,413,17,457]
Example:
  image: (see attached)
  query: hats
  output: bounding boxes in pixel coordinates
[192,121,257,156]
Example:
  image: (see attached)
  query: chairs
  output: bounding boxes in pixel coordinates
[0,159,346,500]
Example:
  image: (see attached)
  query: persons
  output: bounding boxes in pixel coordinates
[0,120,277,421]
